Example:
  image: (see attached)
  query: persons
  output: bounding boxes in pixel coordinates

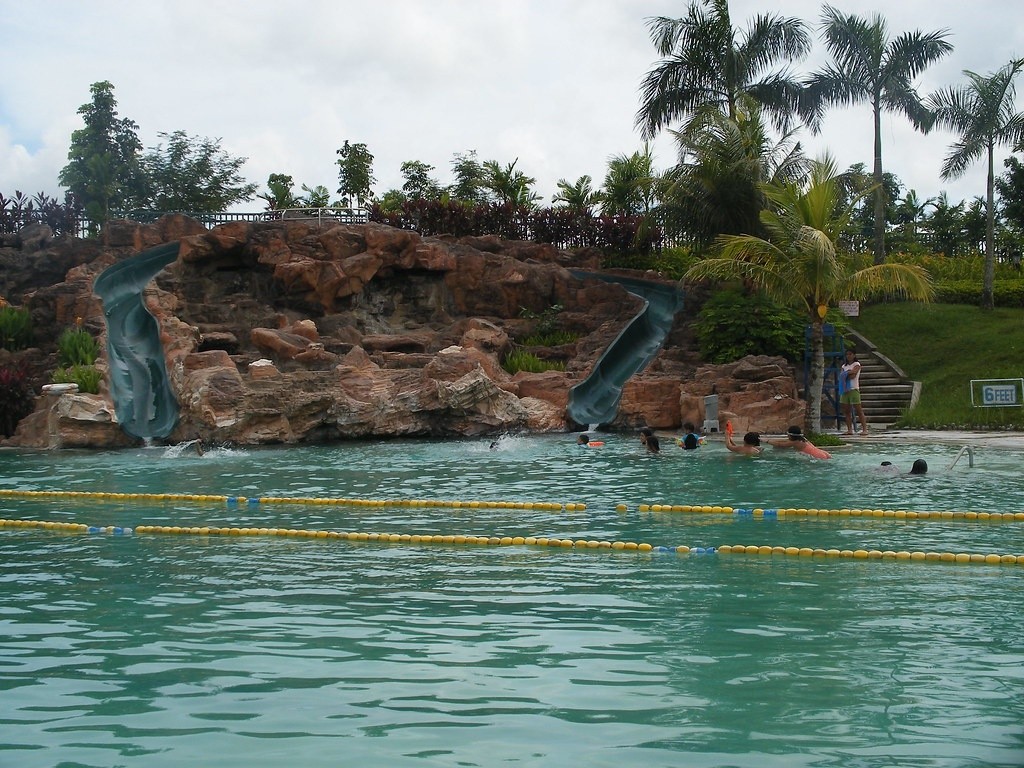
[838,349,869,435]
[645,435,661,453]
[672,421,706,448]
[576,435,590,448]
[759,426,832,458]
[195,439,237,456]
[681,434,700,450]
[910,458,928,475]
[724,420,760,455]
[640,429,652,444]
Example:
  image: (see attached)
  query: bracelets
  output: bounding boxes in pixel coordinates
[767,440,769,443]
